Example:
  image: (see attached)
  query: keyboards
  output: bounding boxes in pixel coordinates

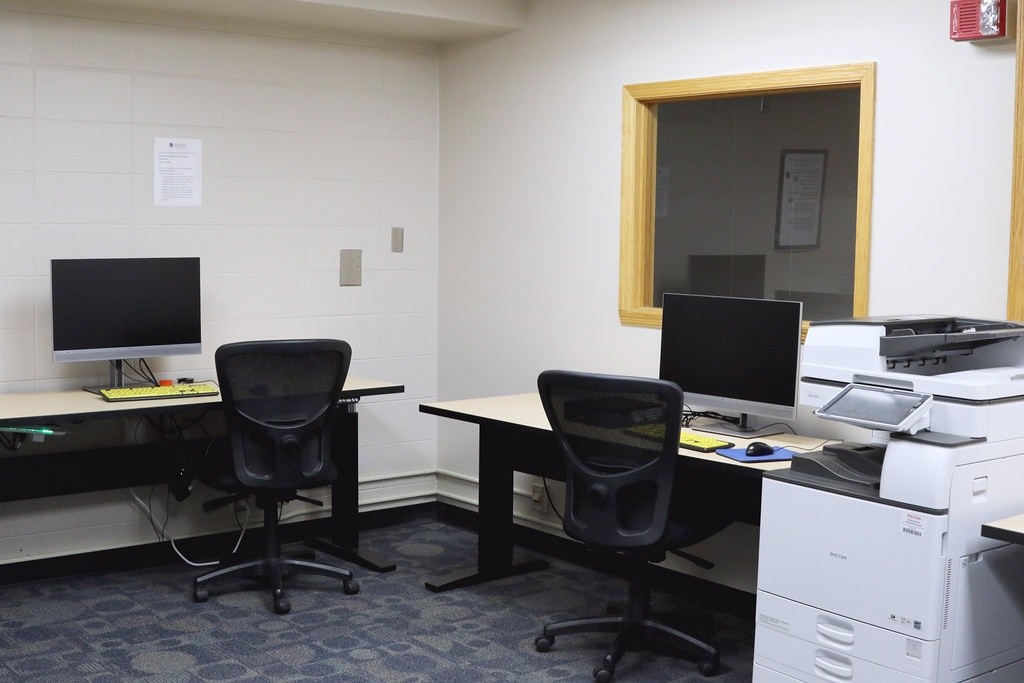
[99,383,219,402]
[623,423,736,452]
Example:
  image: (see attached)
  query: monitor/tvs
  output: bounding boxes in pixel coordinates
[688,255,766,299]
[51,257,202,394]
[659,293,803,438]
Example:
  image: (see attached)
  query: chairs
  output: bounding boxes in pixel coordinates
[197,339,361,613]
[534,368,720,683]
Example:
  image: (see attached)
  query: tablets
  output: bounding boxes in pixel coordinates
[815,383,933,432]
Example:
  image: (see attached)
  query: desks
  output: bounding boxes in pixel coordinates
[0,377,405,567]
[981,514,1024,550]
[418,392,845,592]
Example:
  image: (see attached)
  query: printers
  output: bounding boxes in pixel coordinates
[760,318,1024,683]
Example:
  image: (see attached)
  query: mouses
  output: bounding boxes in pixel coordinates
[249,384,270,395]
[745,442,774,456]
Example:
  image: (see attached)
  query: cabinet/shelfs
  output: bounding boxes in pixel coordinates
[751,478,1024,683]
